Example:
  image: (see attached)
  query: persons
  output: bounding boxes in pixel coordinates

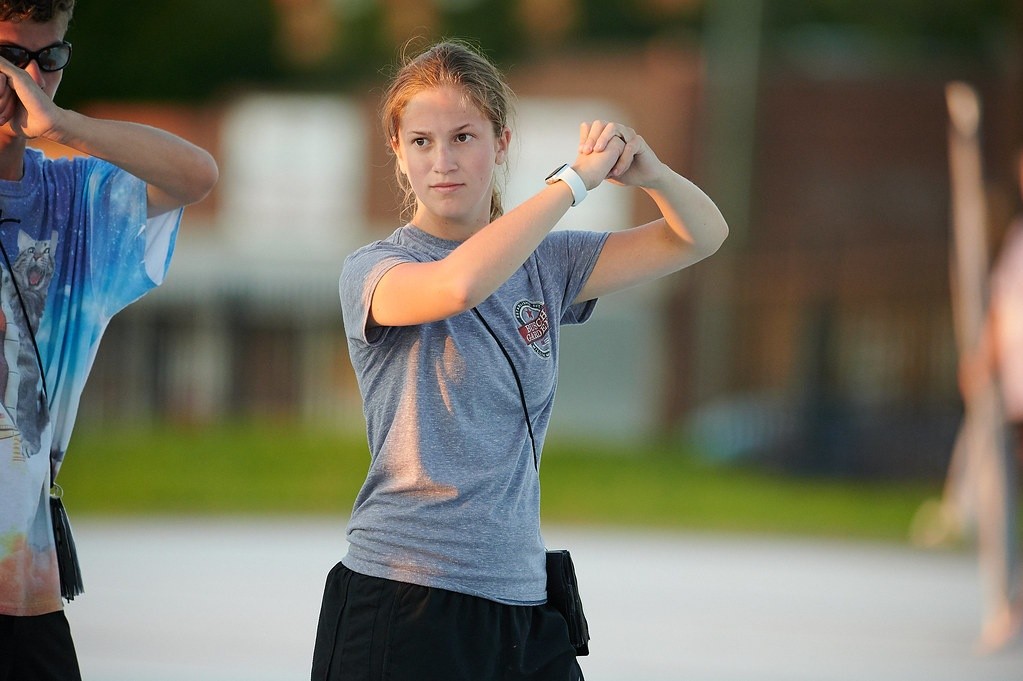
[311,46,731,681]
[0,1,221,681]
[929,82,1023,650]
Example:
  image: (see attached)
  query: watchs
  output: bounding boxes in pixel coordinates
[545,164,588,206]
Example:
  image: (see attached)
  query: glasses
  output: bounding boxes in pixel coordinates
[1,40,72,72]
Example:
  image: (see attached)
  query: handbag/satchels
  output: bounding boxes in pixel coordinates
[543,550,590,656]
